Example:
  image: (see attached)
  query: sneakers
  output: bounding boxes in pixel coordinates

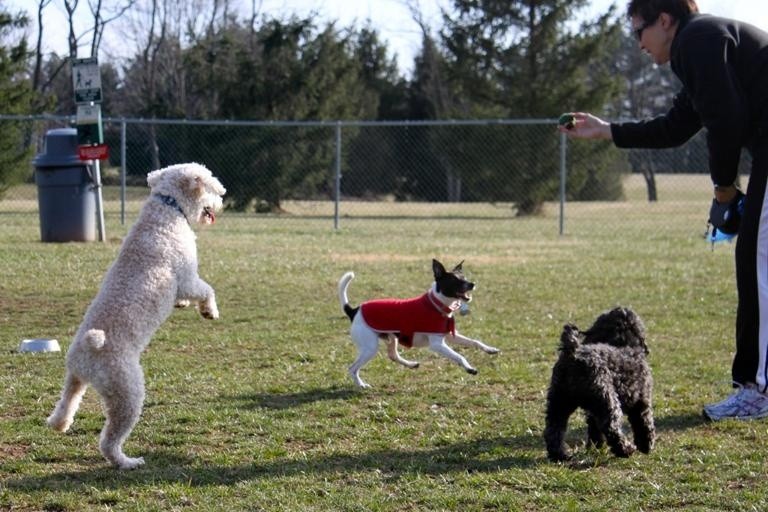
[703,388,768,420]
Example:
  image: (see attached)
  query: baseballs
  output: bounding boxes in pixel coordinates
[559,113,576,130]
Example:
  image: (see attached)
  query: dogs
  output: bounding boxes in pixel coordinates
[335,257,501,394]
[43,158,230,470]
[540,300,658,463]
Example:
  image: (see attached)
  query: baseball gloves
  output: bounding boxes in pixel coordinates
[710,188,746,235]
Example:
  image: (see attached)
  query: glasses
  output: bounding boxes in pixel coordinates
[632,21,650,41]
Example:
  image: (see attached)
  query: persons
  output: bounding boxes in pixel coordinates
[557,0,768,421]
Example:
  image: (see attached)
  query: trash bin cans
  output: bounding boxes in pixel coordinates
[33,126,100,242]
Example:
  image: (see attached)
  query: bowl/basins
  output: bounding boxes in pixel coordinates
[21,339,61,354]
